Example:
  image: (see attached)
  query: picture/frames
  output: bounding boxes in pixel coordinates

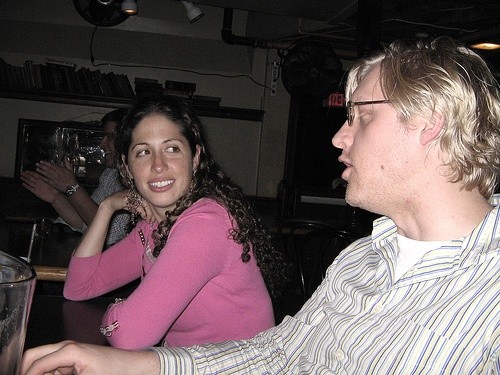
[14,118,105,193]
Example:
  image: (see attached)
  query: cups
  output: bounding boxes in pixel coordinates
[0,248,38,375]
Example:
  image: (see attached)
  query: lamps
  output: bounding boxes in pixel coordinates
[182,0,204,23]
[121,0,138,14]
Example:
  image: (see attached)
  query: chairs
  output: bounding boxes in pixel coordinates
[271,180,369,319]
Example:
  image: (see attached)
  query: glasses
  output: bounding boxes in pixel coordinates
[346,99,399,127]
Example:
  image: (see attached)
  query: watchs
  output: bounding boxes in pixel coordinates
[63,183,80,198]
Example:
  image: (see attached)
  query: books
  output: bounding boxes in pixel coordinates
[0,57,221,108]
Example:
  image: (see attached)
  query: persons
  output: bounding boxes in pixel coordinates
[19,108,138,245]
[15,28,500,373]
[62,92,279,353]
